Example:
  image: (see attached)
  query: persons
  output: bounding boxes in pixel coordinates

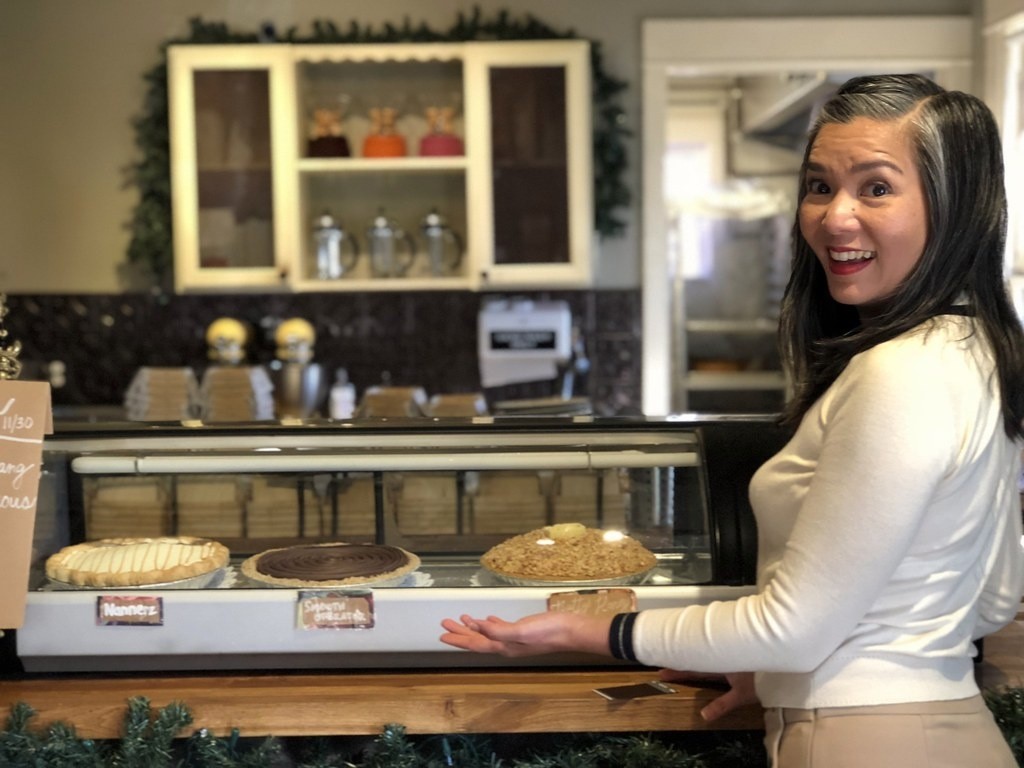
[439,72,1024,768]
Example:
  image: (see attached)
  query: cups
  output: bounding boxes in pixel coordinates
[312,214,362,280]
[419,210,463,276]
[369,215,411,277]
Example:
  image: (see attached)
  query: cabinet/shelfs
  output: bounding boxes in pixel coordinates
[463,41,595,292]
[167,40,295,292]
[291,45,469,288]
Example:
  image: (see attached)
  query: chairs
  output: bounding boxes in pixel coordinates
[695,426,800,585]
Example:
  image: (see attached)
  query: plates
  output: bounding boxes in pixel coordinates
[241,562,419,591]
[481,553,660,586]
[44,563,226,590]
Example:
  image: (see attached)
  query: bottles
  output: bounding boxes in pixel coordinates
[331,367,358,423]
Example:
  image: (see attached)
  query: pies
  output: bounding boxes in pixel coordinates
[46,534,229,586]
